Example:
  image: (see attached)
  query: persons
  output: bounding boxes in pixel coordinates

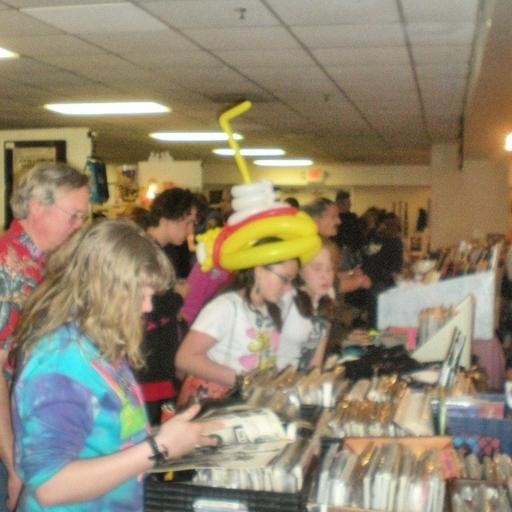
[0,159,401,512]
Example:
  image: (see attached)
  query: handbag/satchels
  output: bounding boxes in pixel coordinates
[176,375,231,413]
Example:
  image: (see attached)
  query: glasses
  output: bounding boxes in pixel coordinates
[55,204,89,226]
[267,268,295,285]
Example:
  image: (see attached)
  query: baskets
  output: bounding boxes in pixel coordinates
[143,397,330,512]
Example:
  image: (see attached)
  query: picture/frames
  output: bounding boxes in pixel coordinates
[4,140,66,231]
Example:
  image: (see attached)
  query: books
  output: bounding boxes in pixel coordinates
[145,364,449,512]
[407,236,498,285]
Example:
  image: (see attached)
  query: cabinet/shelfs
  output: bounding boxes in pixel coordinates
[377,238,503,340]
